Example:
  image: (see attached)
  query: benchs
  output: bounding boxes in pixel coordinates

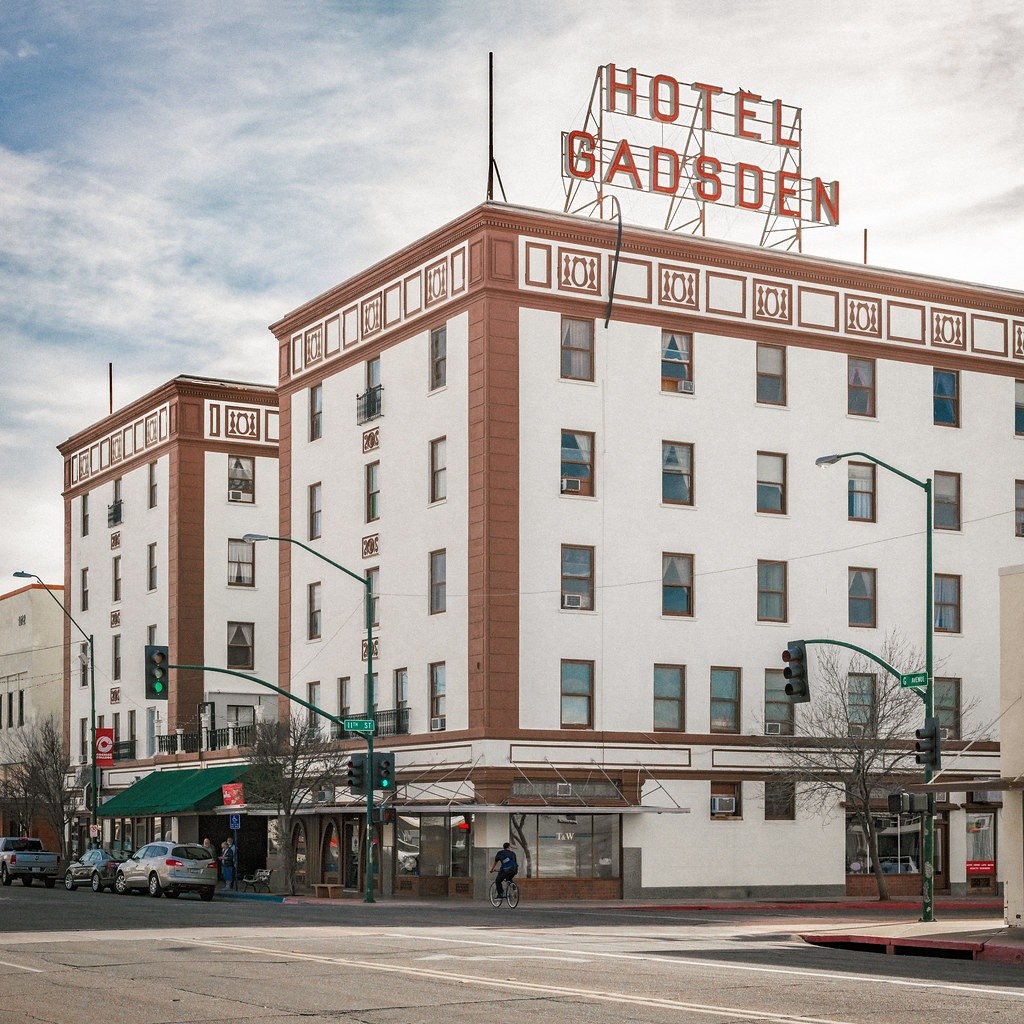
[242,867,274,893]
[310,882,347,900]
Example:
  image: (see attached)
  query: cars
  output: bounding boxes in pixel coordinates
[396,838,419,872]
[113,840,219,901]
[66,850,135,892]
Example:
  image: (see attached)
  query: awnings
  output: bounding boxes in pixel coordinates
[94,763,283,815]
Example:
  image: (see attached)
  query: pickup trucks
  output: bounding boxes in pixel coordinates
[0,837,61,888]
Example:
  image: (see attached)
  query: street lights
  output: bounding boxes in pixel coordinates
[817,450,937,923]
[244,534,377,903]
[12,571,102,847]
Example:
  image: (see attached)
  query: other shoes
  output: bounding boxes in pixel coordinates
[223,887,231,890]
[494,894,503,900]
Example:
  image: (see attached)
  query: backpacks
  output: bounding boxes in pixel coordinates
[502,855,519,878]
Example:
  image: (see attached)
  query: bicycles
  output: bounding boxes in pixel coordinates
[489,870,520,908]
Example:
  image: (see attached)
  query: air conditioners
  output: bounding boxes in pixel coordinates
[712,795,736,813]
[848,724,864,737]
[432,718,446,731]
[561,478,581,492]
[940,726,949,739]
[765,723,781,734]
[228,490,242,501]
[676,380,695,393]
[562,594,582,609]
[78,755,87,764]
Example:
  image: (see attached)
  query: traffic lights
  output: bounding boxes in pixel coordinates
[914,716,942,770]
[888,793,909,813]
[376,752,397,790]
[349,753,373,795]
[145,646,170,701]
[782,639,811,704]
[382,808,399,825]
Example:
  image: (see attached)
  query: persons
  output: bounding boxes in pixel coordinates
[489,843,519,899]
[204,837,237,890]
